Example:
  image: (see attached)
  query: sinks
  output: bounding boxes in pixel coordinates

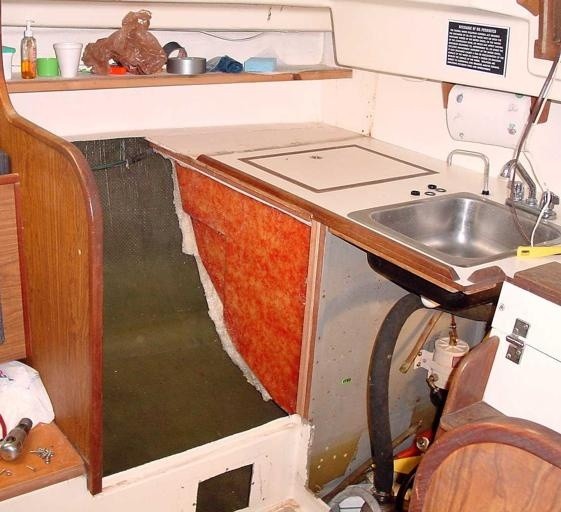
[346,191,561,312]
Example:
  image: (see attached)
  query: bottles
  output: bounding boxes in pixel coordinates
[0,418,33,462]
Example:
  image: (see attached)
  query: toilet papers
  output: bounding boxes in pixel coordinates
[446,85,532,152]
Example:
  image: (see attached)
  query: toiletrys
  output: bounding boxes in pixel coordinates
[20,20,46,81]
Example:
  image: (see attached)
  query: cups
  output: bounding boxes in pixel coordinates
[36,42,82,78]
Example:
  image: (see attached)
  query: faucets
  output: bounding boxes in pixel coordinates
[446,149,491,195]
[498,159,538,207]
